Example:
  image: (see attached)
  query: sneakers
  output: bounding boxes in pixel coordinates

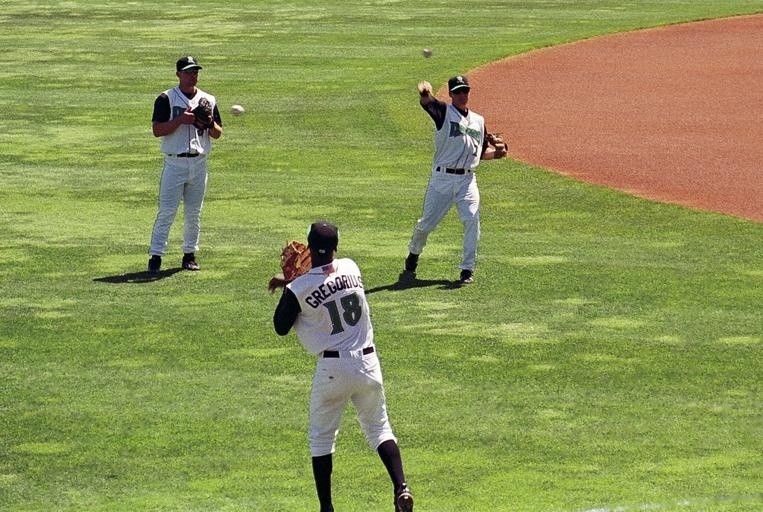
[405,259,418,280]
[393,484,413,511]
[182,256,200,270]
[148,259,161,277]
[460,270,474,283]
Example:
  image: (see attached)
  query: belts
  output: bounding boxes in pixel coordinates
[323,347,374,359]
[168,152,199,158]
[436,166,471,175]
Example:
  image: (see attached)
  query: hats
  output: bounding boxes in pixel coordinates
[176,56,203,73]
[448,76,471,92]
[306,222,339,251]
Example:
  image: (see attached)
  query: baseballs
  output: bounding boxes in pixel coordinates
[231,104,244,116]
[421,48,432,57]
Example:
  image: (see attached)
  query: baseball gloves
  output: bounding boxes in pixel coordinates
[281,240,312,282]
[192,104,214,129]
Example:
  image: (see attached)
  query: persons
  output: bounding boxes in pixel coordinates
[395,75,504,284]
[148,56,222,273]
[270,222,414,511]
